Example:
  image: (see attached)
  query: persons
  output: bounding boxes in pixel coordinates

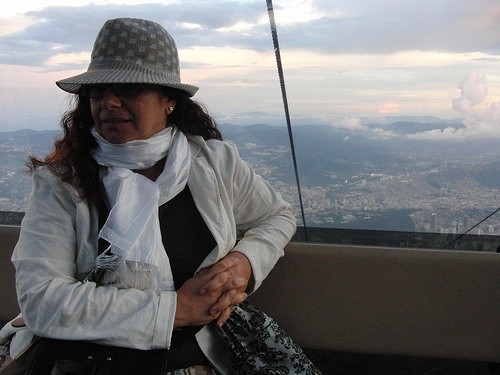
[10,18,297,375]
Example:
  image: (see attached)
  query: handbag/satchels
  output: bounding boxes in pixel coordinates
[209,298,324,375]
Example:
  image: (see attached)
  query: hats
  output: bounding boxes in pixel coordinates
[54,17,200,98]
